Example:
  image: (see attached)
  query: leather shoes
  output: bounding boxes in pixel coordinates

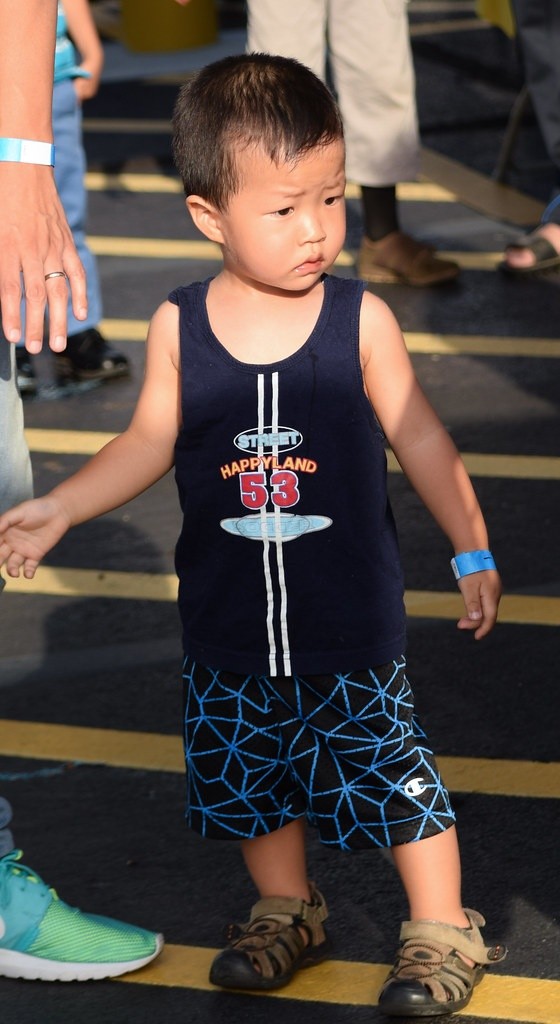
[53,326,128,379]
[16,348,37,394]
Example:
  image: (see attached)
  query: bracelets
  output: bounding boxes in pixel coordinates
[0,138,59,168]
[450,550,497,581]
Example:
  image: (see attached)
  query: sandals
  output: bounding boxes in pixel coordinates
[209,880,332,989]
[378,909,508,1017]
[498,216,560,276]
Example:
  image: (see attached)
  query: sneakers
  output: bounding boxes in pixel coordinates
[0,848,165,983]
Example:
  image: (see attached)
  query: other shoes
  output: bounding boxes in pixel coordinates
[356,235,460,285]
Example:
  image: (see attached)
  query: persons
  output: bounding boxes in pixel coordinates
[0,0,165,982]
[246,0,461,287]
[500,0,560,276]
[0,54,508,1017]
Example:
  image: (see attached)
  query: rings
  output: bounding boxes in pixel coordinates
[45,272,67,279]
